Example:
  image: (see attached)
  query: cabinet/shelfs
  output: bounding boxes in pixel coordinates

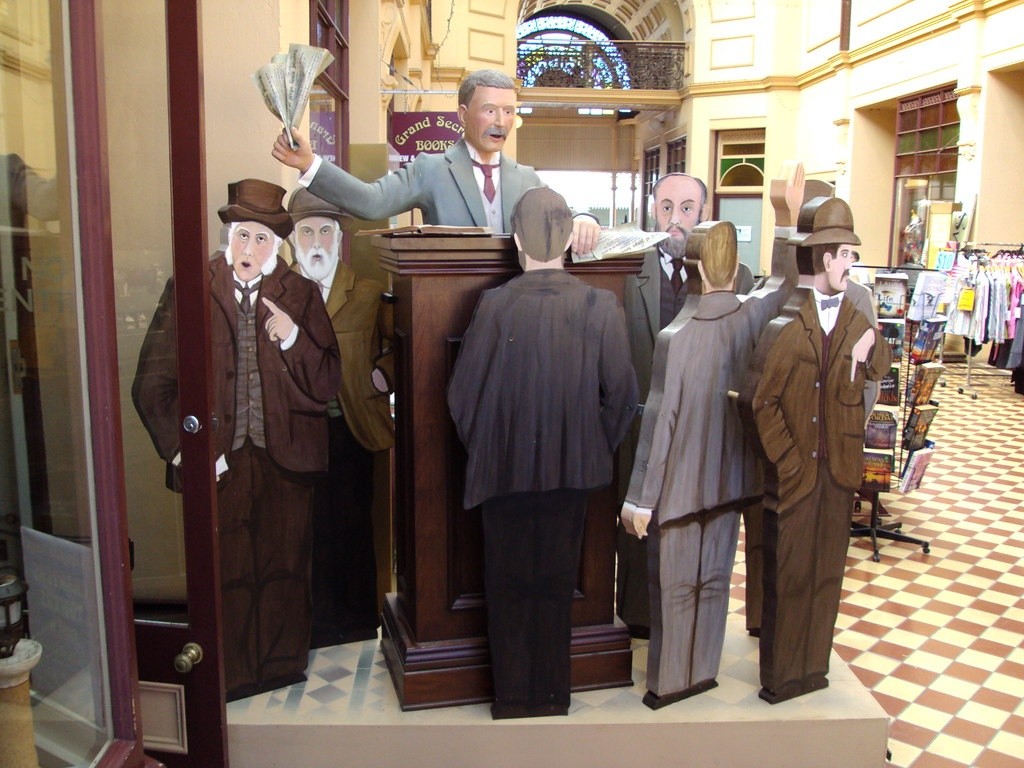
[851,262,952,560]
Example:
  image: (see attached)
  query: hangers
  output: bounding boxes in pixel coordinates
[959,241,1024,269]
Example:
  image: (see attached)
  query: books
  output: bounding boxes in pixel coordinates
[356,223,495,237]
[860,274,949,496]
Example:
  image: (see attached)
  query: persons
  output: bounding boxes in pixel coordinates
[271,70,599,255]
